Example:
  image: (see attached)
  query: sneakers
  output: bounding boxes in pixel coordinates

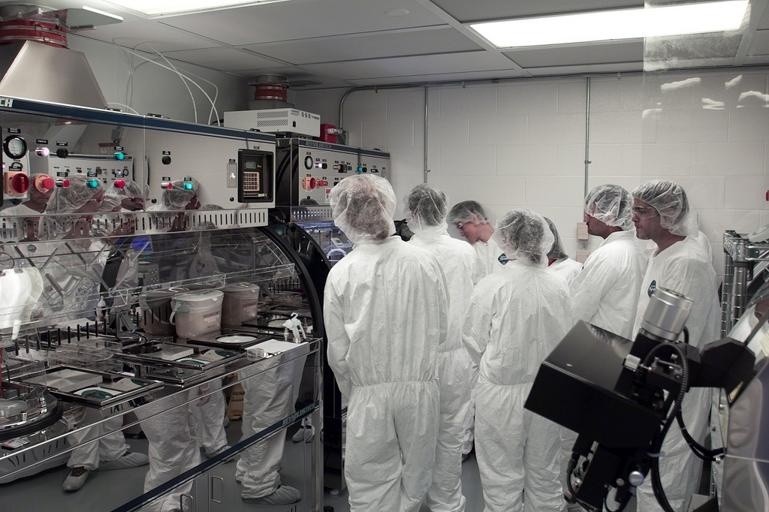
[62,452,149,491]
[292,424,316,442]
[241,485,301,505]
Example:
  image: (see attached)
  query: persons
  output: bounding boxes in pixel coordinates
[323,172,722,512]
[0,172,315,511]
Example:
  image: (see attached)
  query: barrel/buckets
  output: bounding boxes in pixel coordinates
[168,288,224,338]
[220,282,259,326]
